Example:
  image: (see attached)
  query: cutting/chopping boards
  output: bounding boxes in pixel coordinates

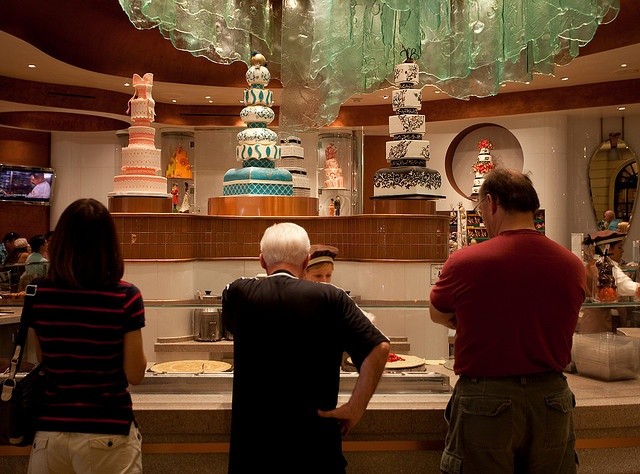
[151,359,232,373]
[347,354,426,369]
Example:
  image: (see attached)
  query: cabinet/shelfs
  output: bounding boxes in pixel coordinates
[436,210,545,257]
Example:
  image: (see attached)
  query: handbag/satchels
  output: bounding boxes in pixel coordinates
[0,276,40,438]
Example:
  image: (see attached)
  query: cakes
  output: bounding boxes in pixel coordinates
[223,48,293,195]
[470,139,495,194]
[325,172,342,188]
[279,135,303,159]
[373,44,441,195]
[289,167,309,187]
[113,72,167,192]
[324,146,340,168]
[167,147,192,178]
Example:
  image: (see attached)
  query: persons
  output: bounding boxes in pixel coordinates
[24,198,147,474]
[583,229,639,308]
[0,231,56,285]
[26,171,52,199]
[429,167,587,474]
[222,222,391,474]
[304,244,339,284]
[602,210,618,231]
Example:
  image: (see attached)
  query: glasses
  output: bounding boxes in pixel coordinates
[473,198,487,216]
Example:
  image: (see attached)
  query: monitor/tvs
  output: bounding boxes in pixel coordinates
[0,163,57,207]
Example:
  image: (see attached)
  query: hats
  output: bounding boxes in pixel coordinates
[590,230,627,246]
[308,244,338,268]
[13,238,30,249]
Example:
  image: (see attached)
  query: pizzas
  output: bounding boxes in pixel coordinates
[346,352,423,371]
[151,360,228,374]
